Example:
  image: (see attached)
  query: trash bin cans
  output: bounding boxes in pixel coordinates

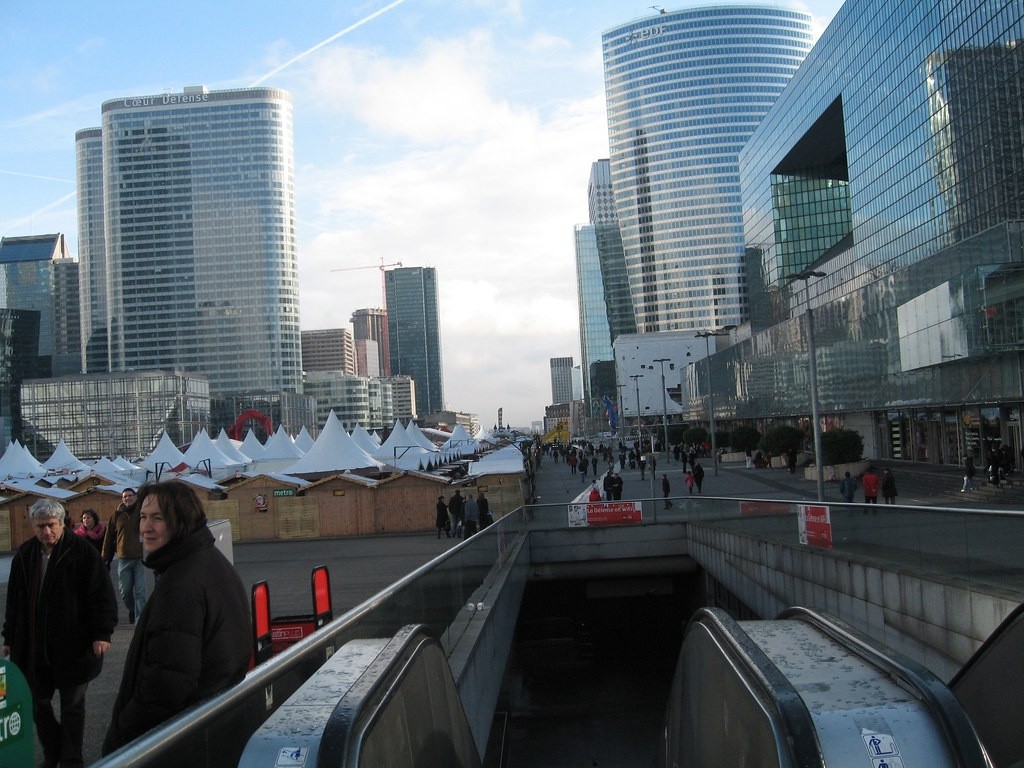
[252,565,335,708]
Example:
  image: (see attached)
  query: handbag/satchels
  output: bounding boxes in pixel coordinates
[444,521,452,531]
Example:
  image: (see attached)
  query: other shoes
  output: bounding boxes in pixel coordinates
[128,609,136,624]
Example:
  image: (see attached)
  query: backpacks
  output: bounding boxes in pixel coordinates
[966,463,976,477]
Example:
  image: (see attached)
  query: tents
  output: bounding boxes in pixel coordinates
[0,409,495,484]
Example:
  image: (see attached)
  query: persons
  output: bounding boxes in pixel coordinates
[603,472,623,501]
[744,446,774,470]
[881,467,898,514]
[862,465,880,516]
[985,442,1014,488]
[592,480,599,492]
[541,438,721,482]
[2,497,120,768]
[662,474,674,510]
[103,482,255,768]
[62,487,148,628]
[436,490,489,540]
[960,454,977,493]
[786,447,797,474]
[684,462,704,495]
[839,471,858,516]
